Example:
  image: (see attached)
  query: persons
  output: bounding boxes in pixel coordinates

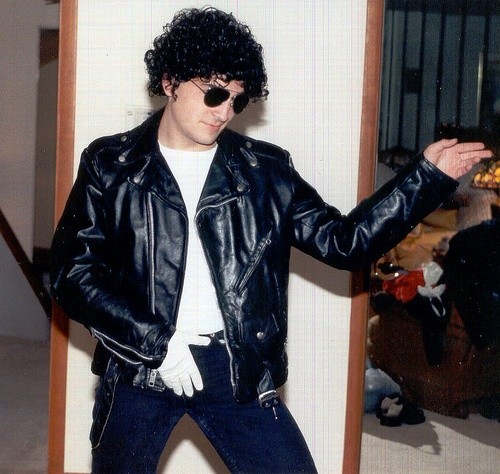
[51,4,493,474]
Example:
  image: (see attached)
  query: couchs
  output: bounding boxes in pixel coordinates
[369,209,500,420]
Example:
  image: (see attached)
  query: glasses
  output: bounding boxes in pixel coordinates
[188,77,250,114]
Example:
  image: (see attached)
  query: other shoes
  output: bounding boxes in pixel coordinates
[375,392,416,418]
[379,403,426,426]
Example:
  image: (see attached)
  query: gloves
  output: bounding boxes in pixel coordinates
[155,331,210,396]
